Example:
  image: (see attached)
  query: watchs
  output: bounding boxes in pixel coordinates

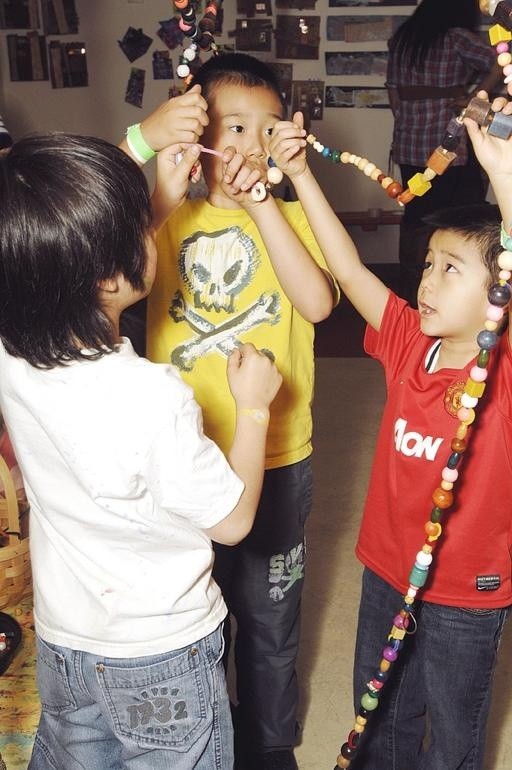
[124,122,157,168]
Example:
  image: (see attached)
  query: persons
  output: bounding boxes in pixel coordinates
[116,51,340,770]
[268,88,512,770]
[0,132,284,770]
[384,0,511,311]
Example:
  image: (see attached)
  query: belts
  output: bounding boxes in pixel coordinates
[396,86,466,101]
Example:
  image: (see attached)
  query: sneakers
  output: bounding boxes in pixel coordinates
[250,748,299,770]
